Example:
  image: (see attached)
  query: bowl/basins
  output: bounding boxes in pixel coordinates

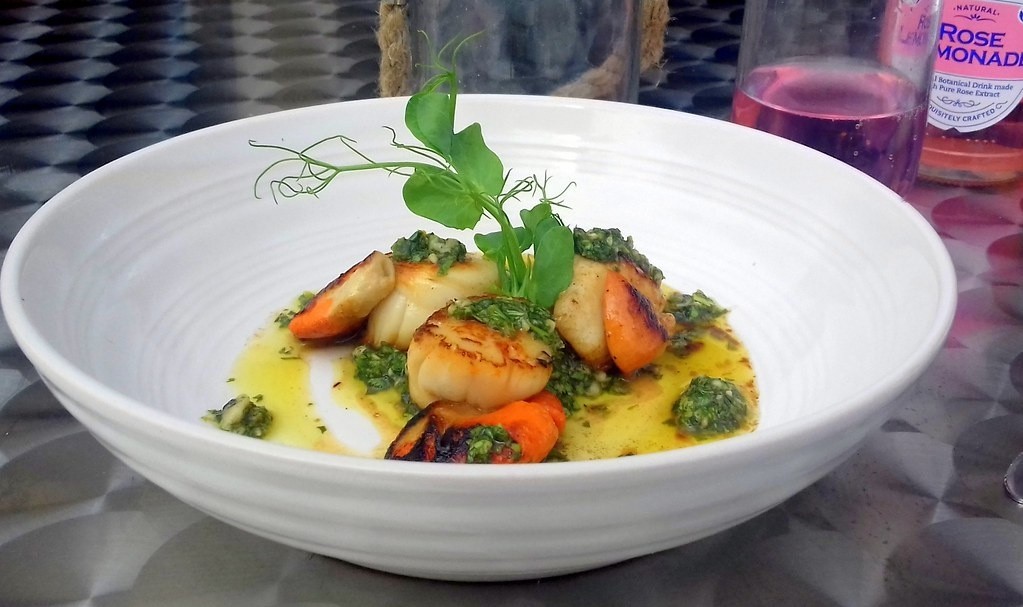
[0,93,958,582]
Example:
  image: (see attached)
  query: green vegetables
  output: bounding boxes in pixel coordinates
[201,27,748,463]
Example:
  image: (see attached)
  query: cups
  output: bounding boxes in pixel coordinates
[880,0,1023,188]
[730,0,944,201]
[403,0,644,104]
